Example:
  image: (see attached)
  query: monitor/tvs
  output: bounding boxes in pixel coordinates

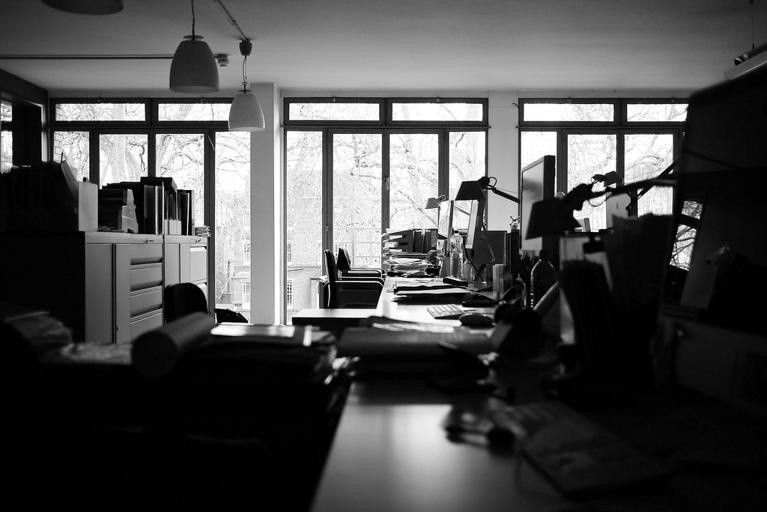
[519,154,557,242]
[463,199,485,250]
[437,199,454,240]
[656,185,766,342]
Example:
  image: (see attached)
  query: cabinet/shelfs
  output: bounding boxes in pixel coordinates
[0,230,208,343]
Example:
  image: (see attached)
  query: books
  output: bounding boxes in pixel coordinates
[103,176,196,238]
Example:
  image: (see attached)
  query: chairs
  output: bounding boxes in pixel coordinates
[325,247,387,310]
[212,307,247,322]
[162,281,207,325]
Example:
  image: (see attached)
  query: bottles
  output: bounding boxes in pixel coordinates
[449,230,464,279]
[528,249,558,306]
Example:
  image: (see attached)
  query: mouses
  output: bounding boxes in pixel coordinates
[459,313,493,327]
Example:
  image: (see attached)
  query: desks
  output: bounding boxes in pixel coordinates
[314,269,696,512]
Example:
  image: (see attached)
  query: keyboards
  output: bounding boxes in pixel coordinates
[427,304,466,319]
[428,276,444,284]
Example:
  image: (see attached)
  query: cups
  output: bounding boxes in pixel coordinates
[143,183,164,235]
[468,263,487,292]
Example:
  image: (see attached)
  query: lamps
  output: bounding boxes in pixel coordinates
[426,194,496,265]
[227,38,265,131]
[455,176,520,203]
[169,1,220,93]
[526,170,625,241]
